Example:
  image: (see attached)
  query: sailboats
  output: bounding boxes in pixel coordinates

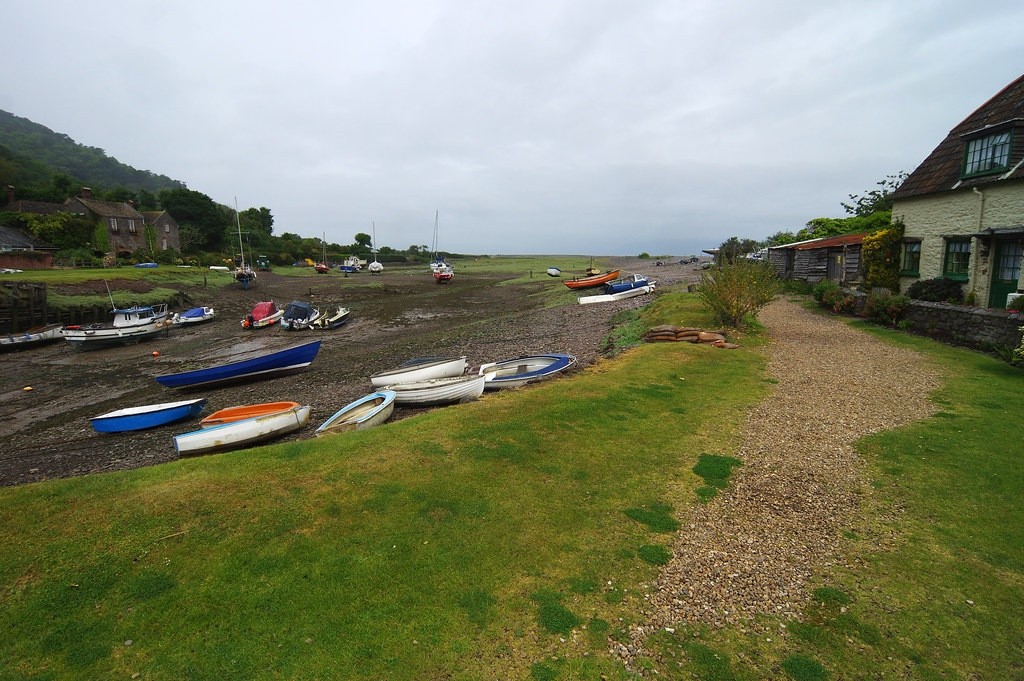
[368,221,384,273]
[229,196,257,290]
[429,210,455,285]
[313,232,330,274]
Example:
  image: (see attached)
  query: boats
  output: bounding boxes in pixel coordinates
[86,398,206,434]
[61,278,215,351]
[338,253,367,273]
[240,299,352,332]
[172,401,312,456]
[314,389,397,437]
[154,337,324,395]
[369,355,485,407]
[0,321,68,350]
[467,352,580,390]
[562,256,657,305]
